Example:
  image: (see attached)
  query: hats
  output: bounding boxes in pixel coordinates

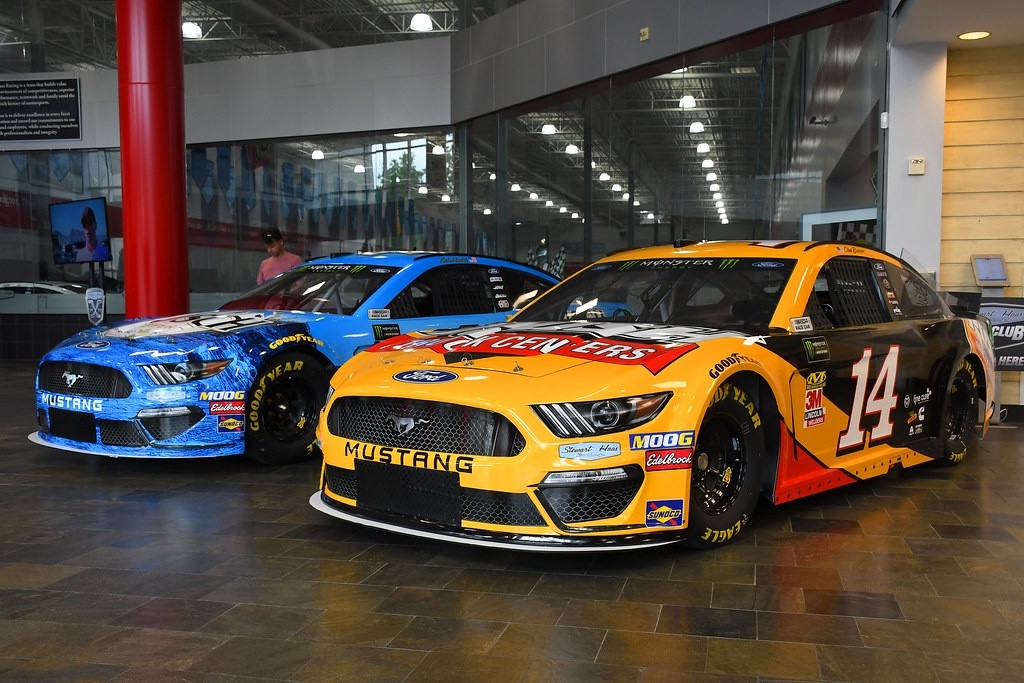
[263,227,282,240]
[81,207,96,225]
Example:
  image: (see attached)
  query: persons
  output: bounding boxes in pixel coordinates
[257,228,306,310]
[75,208,110,261]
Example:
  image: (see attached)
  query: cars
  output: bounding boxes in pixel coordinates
[0,281,90,294]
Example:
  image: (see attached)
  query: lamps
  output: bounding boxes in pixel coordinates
[311,150,324,160]
[418,187,428,194]
[510,181,586,244]
[541,125,557,134]
[410,13,433,31]
[431,145,445,155]
[483,208,491,215]
[592,69,729,226]
[441,194,451,202]
[353,165,365,173]
[565,144,579,154]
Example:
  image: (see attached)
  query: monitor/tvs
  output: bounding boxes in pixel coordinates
[48,196,113,264]
[970,253,1010,287]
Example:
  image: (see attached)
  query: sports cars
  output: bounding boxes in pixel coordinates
[308,241,997,554]
[27,250,623,459]
